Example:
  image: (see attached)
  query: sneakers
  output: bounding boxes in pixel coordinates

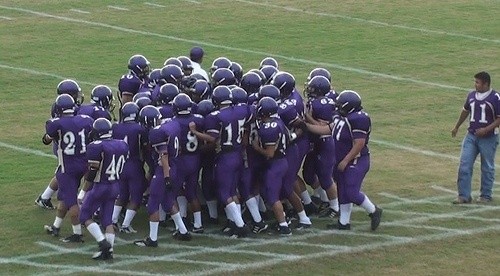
[43,224,61,237]
[76,198,139,234]
[141,191,316,239]
[171,230,192,240]
[327,220,350,230]
[91,241,113,259]
[63,234,84,243]
[310,195,321,205]
[134,236,158,248]
[319,202,330,210]
[318,208,340,219]
[34,196,56,209]
[367,206,383,232]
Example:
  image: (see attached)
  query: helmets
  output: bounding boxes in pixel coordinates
[55,54,364,138]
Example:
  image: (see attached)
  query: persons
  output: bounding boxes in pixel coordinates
[451,71,500,205]
[33,46,383,259]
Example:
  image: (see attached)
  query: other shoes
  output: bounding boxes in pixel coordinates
[453,197,465,204]
[477,196,490,203]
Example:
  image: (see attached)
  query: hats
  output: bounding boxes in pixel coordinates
[189,47,203,59]
